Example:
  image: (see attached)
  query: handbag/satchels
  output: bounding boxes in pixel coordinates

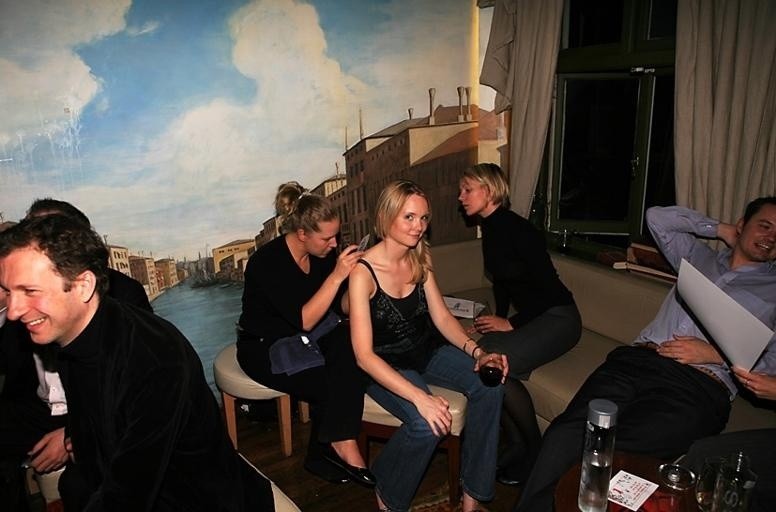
[270,333,326,377]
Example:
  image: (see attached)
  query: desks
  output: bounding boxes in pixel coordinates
[553,446,705,512]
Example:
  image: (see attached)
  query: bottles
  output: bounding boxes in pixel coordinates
[638,461,696,512]
[712,444,762,512]
[473,300,492,335]
[577,397,620,512]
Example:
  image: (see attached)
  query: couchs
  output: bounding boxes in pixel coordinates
[423,238,776,438]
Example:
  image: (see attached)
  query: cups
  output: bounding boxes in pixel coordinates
[694,460,724,511]
[480,349,505,387]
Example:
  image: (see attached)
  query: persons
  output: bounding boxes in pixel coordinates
[730,366,776,401]
[1,195,154,512]
[335,178,509,511]
[233,180,376,492]
[2,211,277,511]
[515,195,776,510]
[459,161,581,486]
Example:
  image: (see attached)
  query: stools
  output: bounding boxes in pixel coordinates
[214,343,311,457]
[361,384,469,506]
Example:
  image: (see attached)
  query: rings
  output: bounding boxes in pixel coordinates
[746,379,751,386]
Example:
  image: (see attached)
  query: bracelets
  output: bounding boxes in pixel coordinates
[472,345,481,359]
[463,337,475,353]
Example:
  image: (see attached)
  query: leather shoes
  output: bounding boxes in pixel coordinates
[496,456,531,486]
[316,441,377,486]
[303,456,352,486]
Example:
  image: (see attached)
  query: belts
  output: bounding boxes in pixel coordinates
[637,340,723,384]
